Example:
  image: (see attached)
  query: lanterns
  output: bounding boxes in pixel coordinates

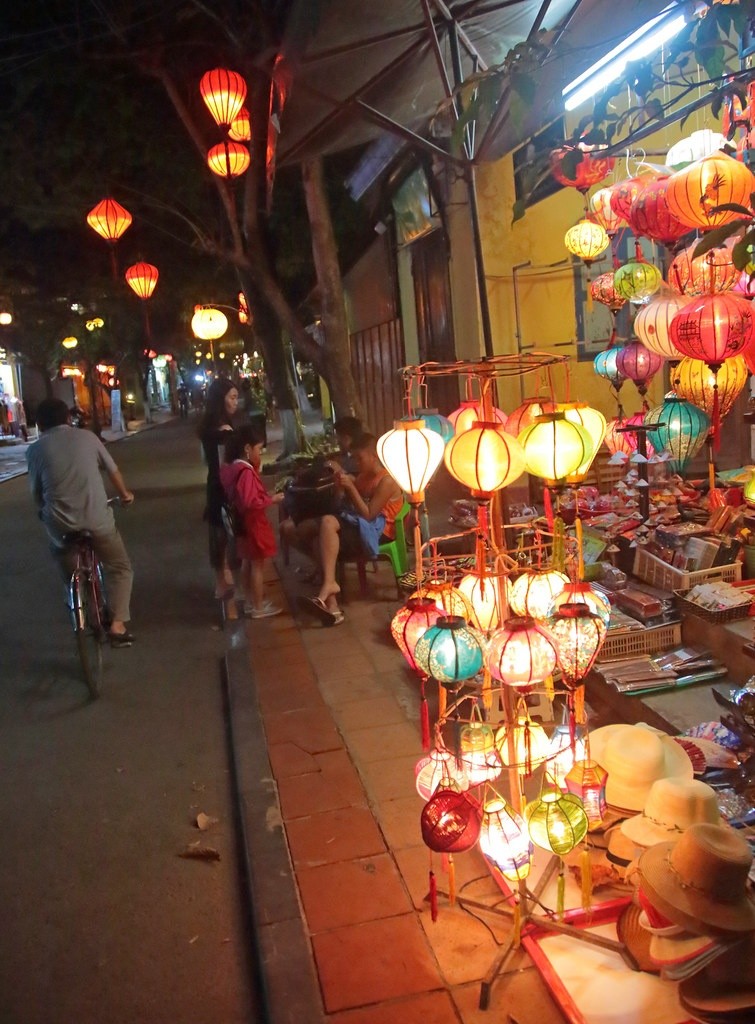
[374,122,754,954]
[50,65,267,383]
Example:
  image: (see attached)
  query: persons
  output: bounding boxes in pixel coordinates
[294,436,405,627]
[219,424,285,618]
[176,382,189,416]
[25,398,143,642]
[196,377,245,597]
[275,415,373,584]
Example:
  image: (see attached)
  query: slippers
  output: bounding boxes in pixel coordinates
[329,608,345,626]
[295,595,335,626]
[107,628,136,641]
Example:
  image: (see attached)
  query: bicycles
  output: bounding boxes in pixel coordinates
[38,496,136,700]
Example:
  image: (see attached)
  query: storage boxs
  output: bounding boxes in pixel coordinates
[633,544,744,592]
[595,617,683,665]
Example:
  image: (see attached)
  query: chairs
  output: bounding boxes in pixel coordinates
[334,502,412,593]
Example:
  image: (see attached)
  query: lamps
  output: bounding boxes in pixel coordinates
[559,4,696,113]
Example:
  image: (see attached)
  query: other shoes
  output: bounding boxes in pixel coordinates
[215,584,240,600]
[296,565,325,587]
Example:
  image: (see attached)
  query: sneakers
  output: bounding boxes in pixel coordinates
[243,599,271,615]
[250,605,284,619]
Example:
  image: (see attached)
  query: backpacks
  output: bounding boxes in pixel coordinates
[220,467,252,539]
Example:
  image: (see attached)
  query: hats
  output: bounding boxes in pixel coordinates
[561,719,755,1024]
